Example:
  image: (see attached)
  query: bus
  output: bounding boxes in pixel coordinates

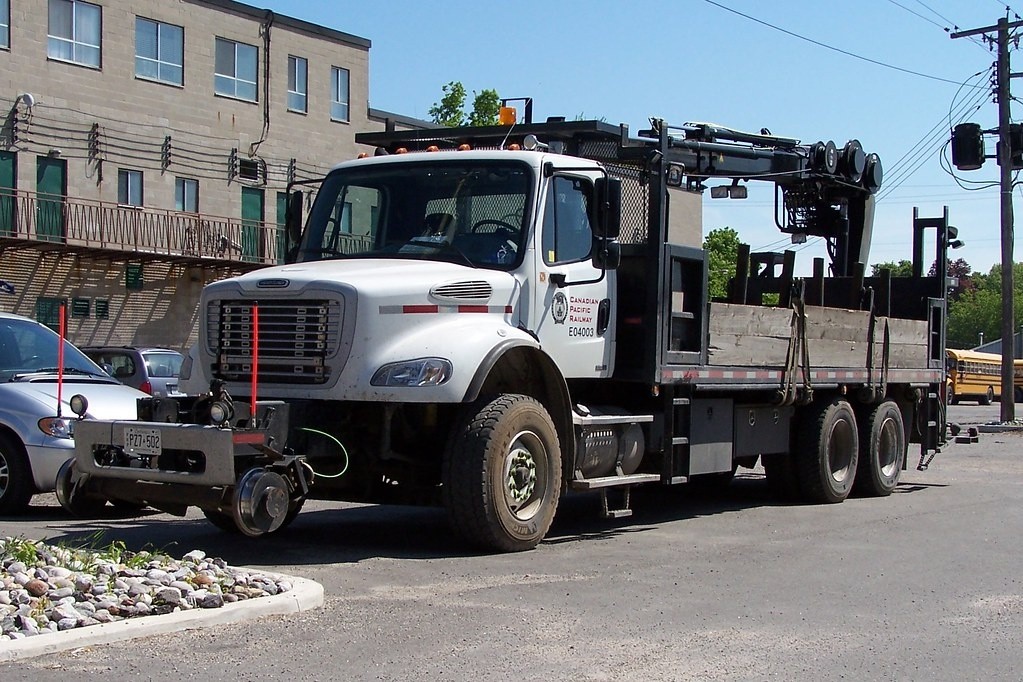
[945,347,1023,406]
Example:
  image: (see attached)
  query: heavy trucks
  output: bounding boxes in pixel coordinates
[54,121,953,553]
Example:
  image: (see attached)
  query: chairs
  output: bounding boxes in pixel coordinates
[552,203,574,228]
[424,212,452,236]
[155,366,171,377]
[114,367,129,377]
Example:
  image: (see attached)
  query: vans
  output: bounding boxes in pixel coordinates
[78,347,185,401]
[1,310,156,518]
